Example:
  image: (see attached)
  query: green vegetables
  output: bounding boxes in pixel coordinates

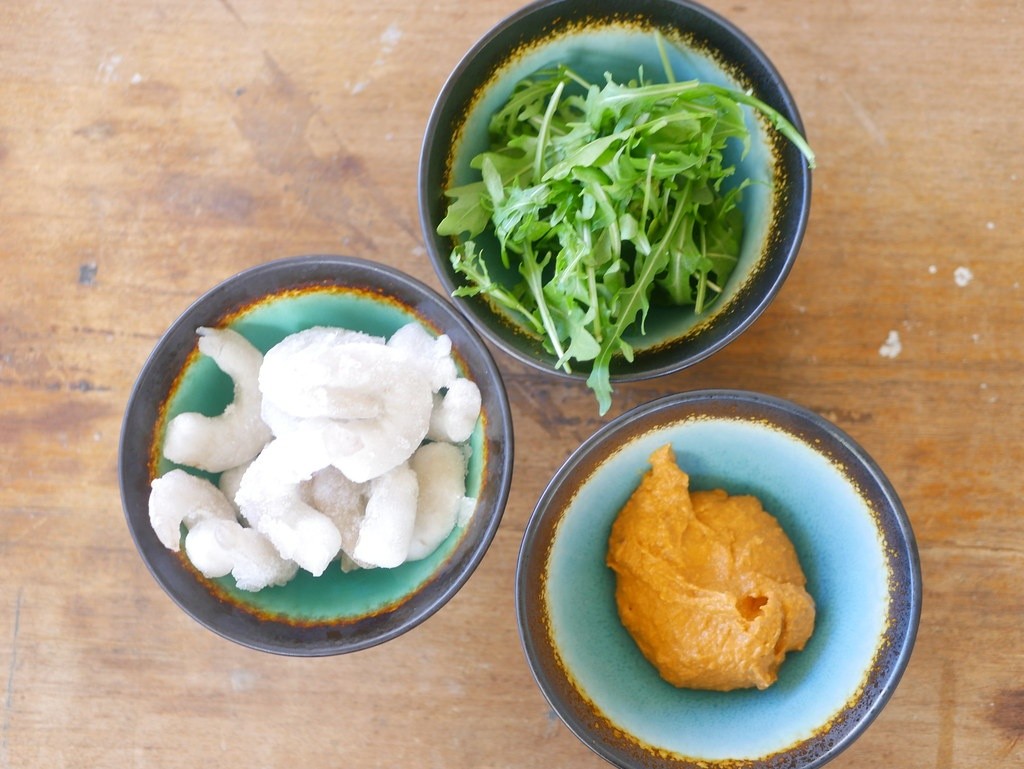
[434,29,816,417]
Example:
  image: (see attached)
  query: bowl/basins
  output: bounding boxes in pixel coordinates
[417,1,814,381]
[116,256,513,659]
[515,390,924,769]
